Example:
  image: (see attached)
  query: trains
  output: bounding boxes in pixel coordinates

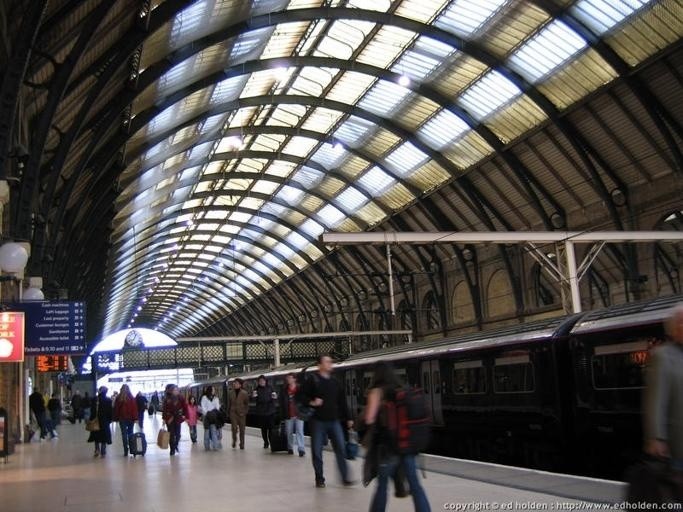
[140,291,682,508]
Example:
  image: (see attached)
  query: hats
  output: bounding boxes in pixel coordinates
[256,375,268,382]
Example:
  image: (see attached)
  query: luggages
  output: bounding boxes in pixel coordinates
[130,422,147,456]
[271,426,289,452]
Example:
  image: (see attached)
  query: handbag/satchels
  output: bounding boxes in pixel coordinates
[346,426,359,460]
[157,424,170,449]
[294,398,316,420]
[85,418,100,430]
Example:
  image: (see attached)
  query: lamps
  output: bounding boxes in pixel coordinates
[20,285,47,301]
[0,237,28,281]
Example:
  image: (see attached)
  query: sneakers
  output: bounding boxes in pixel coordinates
[315,477,327,488]
[340,478,361,487]
[232,443,244,450]
[93,448,105,458]
[39,435,59,441]
[263,442,270,449]
[288,448,306,457]
[122,447,129,456]
[193,441,220,451]
[170,449,176,455]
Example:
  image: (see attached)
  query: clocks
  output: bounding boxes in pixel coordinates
[123,329,143,348]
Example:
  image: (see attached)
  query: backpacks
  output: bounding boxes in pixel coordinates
[388,387,430,447]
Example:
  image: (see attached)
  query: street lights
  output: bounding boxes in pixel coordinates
[19,285,44,302]
[66,356,78,378]
[0,242,22,444]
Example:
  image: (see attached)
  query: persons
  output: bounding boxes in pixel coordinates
[643,306,683,459]
